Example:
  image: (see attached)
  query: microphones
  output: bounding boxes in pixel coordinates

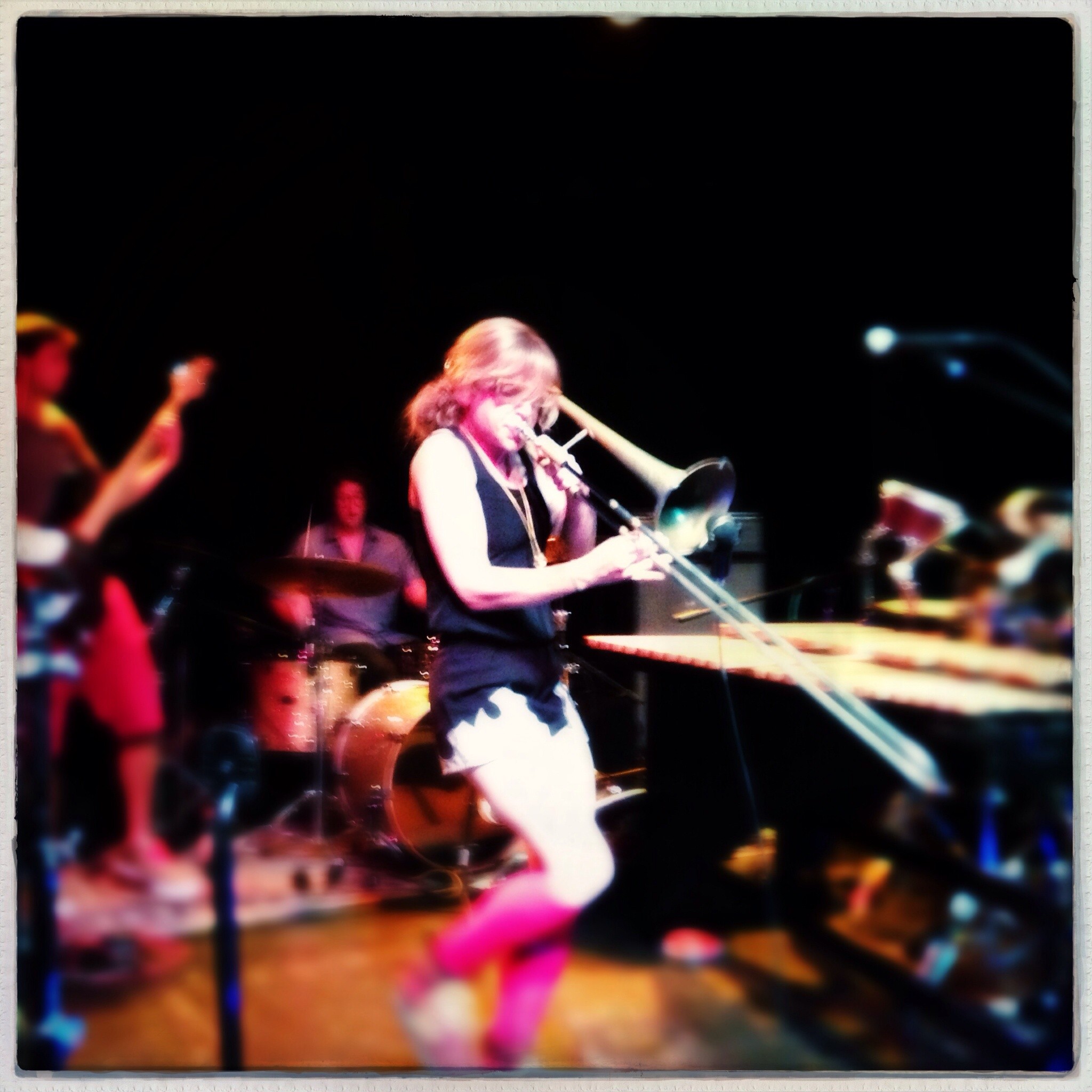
[864,327,983,356]
[707,512,738,591]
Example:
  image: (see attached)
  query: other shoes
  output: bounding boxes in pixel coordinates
[392,973,482,1068]
[101,844,200,895]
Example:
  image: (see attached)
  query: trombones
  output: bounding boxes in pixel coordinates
[521,387,957,798]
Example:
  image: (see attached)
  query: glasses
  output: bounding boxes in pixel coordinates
[481,378,549,421]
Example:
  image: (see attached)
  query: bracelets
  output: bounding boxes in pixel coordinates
[571,559,585,590]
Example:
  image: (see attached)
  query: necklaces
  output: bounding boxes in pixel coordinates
[460,426,548,569]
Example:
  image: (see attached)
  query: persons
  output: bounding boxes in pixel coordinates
[16,312,207,902]
[270,478,427,649]
[398,318,674,1067]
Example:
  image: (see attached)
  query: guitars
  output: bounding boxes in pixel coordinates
[17,355,217,659]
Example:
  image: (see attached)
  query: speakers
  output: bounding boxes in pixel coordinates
[632,509,764,638]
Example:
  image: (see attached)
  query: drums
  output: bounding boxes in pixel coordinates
[250,651,524,878]
